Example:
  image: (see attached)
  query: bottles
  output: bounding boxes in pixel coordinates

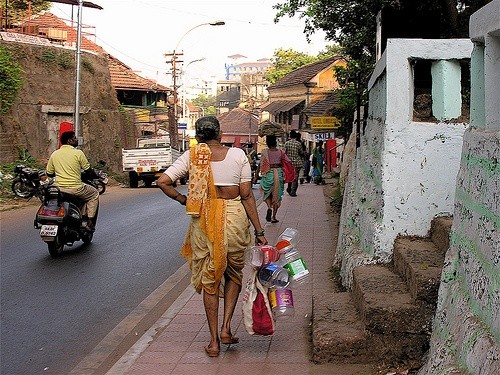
[267,287,294,322]
[258,264,291,289]
[245,245,281,266]
[278,248,310,286]
[274,228,301,254]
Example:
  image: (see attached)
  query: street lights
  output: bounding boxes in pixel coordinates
[172,19,227,150]
[182,58,206,153]
[235,81,252,144]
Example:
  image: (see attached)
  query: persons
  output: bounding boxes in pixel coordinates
[241,142,260,184]
[45,131,102,230]
[294,132,326,185]
[283,131,304,197]
[258,135,299,223]
[157,116,269,357]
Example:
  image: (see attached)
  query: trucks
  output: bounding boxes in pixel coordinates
[121,133,187,188]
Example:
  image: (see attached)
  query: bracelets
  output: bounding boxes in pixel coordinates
[254,229,265,236]
[172,193,181,199]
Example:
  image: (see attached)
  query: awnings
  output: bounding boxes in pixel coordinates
[260,100,304,115]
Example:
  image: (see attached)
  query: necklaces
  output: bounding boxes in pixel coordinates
[207,144,222,146]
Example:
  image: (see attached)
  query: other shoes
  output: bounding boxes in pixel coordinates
[85,218,95,230]
[221,333,240,343]
[265,209,279,223]
[286,188,297,197]
[203,342,220,357]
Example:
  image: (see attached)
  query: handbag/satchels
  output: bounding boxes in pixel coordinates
[241,270,275,338]
[280,149,295,182]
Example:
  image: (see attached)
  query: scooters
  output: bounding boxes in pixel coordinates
[250,152,262,184]
[80,166,109,195]
[34,160,107,259]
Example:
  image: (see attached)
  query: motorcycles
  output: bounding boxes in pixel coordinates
[11,149,50,203]
